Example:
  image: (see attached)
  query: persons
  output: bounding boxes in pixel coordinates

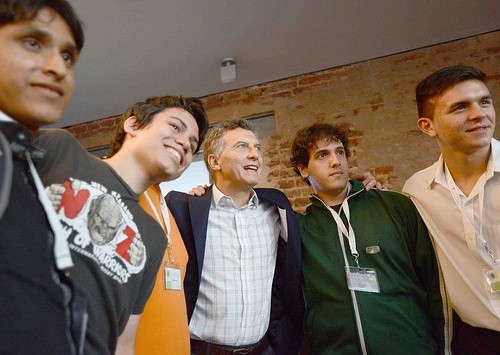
[400,63,500,355]
[164,118,385,353]
[186,122,453,355]
[133,183,192,355]
[31,94,209,355]
[0,0,88,355]
